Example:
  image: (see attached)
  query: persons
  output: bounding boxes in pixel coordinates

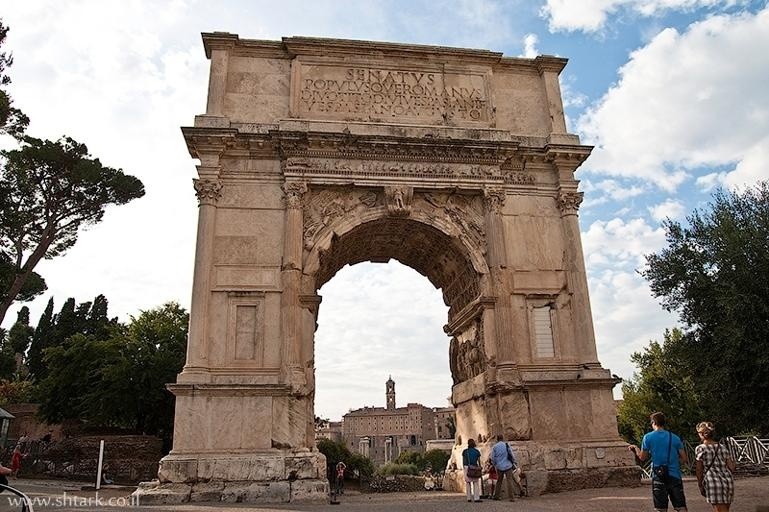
[100,464,114,485]
[628,412,689,512]
[336,462,347,494]
[461,435,525,502]
[694,421,736,511]
[0,429,55,494]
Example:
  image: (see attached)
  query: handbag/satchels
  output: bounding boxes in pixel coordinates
[698,475,706,497]
[653,465,668,483]
[467,465,482,478]
